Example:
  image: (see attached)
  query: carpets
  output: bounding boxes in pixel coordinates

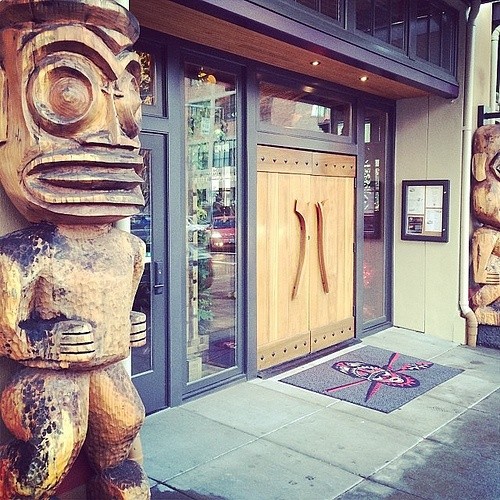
[278,344,464,415]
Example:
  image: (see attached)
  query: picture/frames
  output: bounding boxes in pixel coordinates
[401,180,449,242]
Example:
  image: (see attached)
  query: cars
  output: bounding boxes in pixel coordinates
[186,216,209,241]
[208,216,236,252]
[138,242,212,307]
